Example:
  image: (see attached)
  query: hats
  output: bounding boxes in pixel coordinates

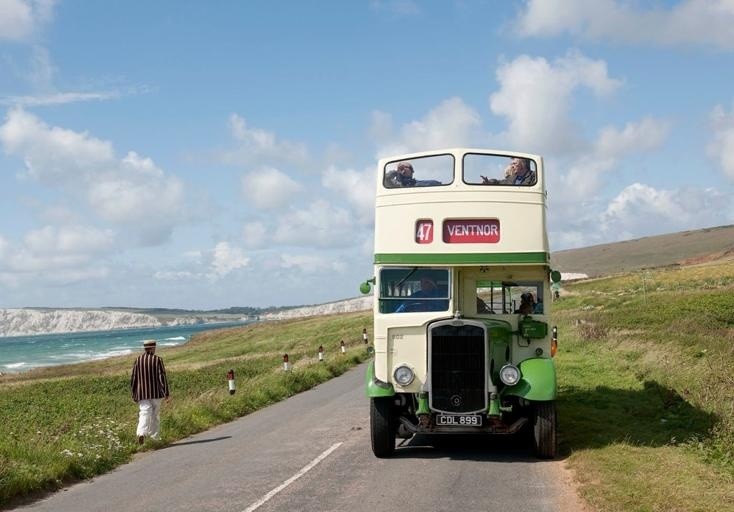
[143,340,156,349]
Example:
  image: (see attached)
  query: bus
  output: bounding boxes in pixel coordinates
[356,142,563,463]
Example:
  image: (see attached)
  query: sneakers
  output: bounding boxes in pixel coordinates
[138,433,145,446]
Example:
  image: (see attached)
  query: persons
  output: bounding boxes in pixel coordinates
[129,340,168,442]
[505,163,514,176]
[480,156,536,185]
[385,162,444,186]
[393,276,447,312]
[514,292,535,314]
